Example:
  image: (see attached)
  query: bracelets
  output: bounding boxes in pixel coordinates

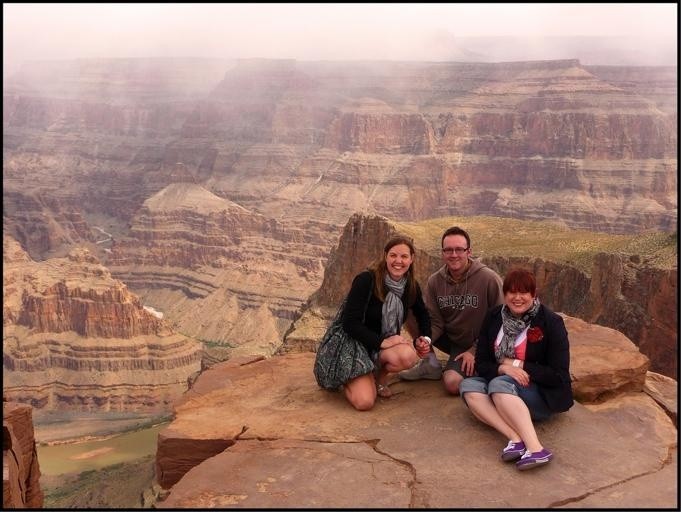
[424,336,431,344]
[512,357,522,368]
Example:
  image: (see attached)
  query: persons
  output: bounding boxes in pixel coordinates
[395,226,505,395]
[458,269,574,472]
[310,239,432,412]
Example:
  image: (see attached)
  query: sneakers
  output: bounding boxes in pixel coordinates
[501,441,526,461]
[398,357,442,381]
[516,450,552,470]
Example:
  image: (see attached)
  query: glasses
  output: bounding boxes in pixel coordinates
[442,248,468,254]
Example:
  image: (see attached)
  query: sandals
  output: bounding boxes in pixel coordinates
[377,382,392,398]
